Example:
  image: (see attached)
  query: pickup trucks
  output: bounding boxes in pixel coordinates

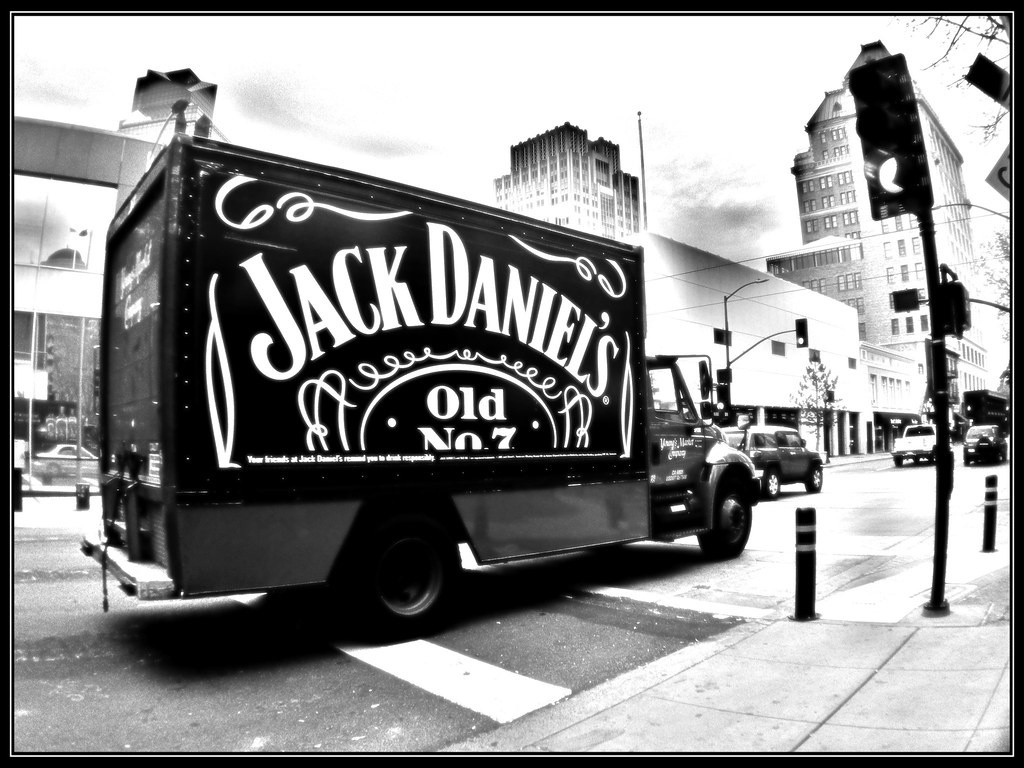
[890,424,954,467]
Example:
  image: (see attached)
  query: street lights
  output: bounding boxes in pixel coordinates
[916,203,1010,222]
[724,278,770,404]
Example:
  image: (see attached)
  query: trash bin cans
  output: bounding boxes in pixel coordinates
[76,483,91,511]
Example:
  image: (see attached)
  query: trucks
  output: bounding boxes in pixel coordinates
[80,132,764,643]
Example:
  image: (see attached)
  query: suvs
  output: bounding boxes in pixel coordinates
[715,426,824,498]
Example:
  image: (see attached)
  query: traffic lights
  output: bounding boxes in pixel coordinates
[795,319,808,349]
[847,53,936,222]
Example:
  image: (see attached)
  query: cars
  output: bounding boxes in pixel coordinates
[29,444,100,478]
[963,425,1008,467]
